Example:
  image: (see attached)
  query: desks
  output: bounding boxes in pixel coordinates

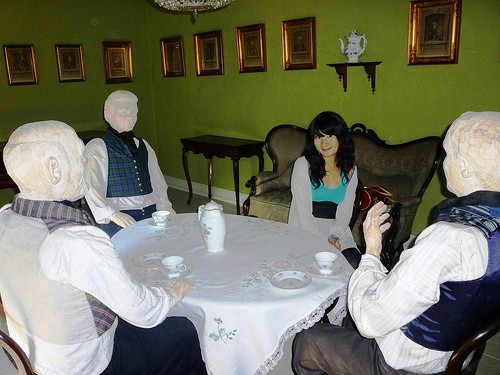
[0,130,107,190]
[110,212,355,375]
[181,134,266,216]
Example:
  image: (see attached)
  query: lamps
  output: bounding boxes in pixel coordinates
[152,0,235,23]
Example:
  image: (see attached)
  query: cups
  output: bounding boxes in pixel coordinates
[160,255,186,277]
[152,210,170,226]
[314,252,338,275]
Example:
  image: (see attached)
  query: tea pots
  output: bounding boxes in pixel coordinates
[338,28,367,63]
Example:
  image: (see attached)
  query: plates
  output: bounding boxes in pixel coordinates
[270,270,312,290]
[149,217,176,228]
[306,262,346,278]
[132,252,166,270]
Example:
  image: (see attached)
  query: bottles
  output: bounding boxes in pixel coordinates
[198,200,226,252]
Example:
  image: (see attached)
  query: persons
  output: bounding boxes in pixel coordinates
[291,111,500,375]
[0,121,206,375]
[288,111,362,269]
[83,90,177,240]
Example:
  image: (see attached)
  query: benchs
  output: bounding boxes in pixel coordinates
[241,123,443,275]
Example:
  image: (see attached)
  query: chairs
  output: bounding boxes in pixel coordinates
[0,330,35,375]
[444,320,500,375]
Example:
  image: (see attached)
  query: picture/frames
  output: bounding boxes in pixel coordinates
[159,35,187,78]
[102,41,134,85]
[235,23,268,74]
[2,44,41,86]
[192,29,225,77]
[280,16,318,71]
[55,44,87,83]
[406,0,462,66]
